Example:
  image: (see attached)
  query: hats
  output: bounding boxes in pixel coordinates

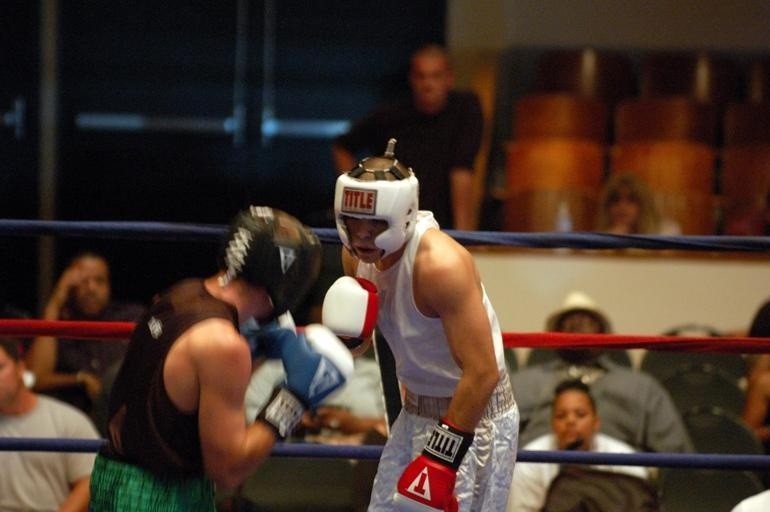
[544,293,614,336]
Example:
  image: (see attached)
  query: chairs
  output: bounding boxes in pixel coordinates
[503,92,769,237]
[636,321,767,510]
[236,441,357,512]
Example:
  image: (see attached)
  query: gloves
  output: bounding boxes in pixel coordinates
[391,415,474,512]
[253,323,354,442]
[322,275,380,351]
[239,308,295,359]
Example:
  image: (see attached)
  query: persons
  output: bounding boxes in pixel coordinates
[319,138,522,511]
[593,170,681,234]
[0,252,769,512]
[713,188,770,236]
[88,203,356,510]
[330,42,485,229]
